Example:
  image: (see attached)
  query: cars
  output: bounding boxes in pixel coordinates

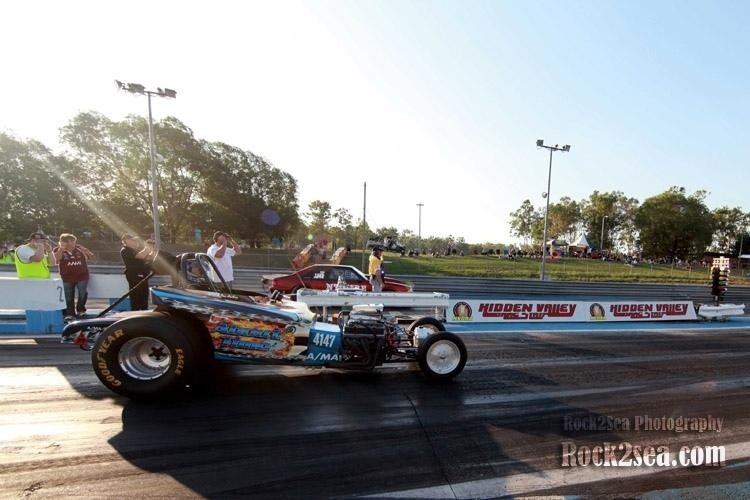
[365,236,406,253]
[663,255,672,263]
[255,261,418,294]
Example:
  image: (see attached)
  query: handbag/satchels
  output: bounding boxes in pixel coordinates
[372,274,377,280]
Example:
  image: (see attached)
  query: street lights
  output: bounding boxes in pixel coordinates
[416,203,425,250]
[112,77,180,249]
[600,215,610,250]
[534,137,572,281]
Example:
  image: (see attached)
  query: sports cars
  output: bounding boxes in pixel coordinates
[55,249,471,403]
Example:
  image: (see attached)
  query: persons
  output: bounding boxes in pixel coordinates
[386,235,583,260]
[0,245,15,263]
[583,248,706,266]
[369,246,385,292]
[15,233,95,320]
[207,231,240,293]
[120,233,154,310]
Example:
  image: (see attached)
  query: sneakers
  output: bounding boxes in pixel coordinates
[63,310,88,323]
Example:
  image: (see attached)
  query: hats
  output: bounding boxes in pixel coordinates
[26,232,49,242]
[373,244,385,251]
[59,233,77,242]
[121,233,139,241]
[213,231,224,241]
